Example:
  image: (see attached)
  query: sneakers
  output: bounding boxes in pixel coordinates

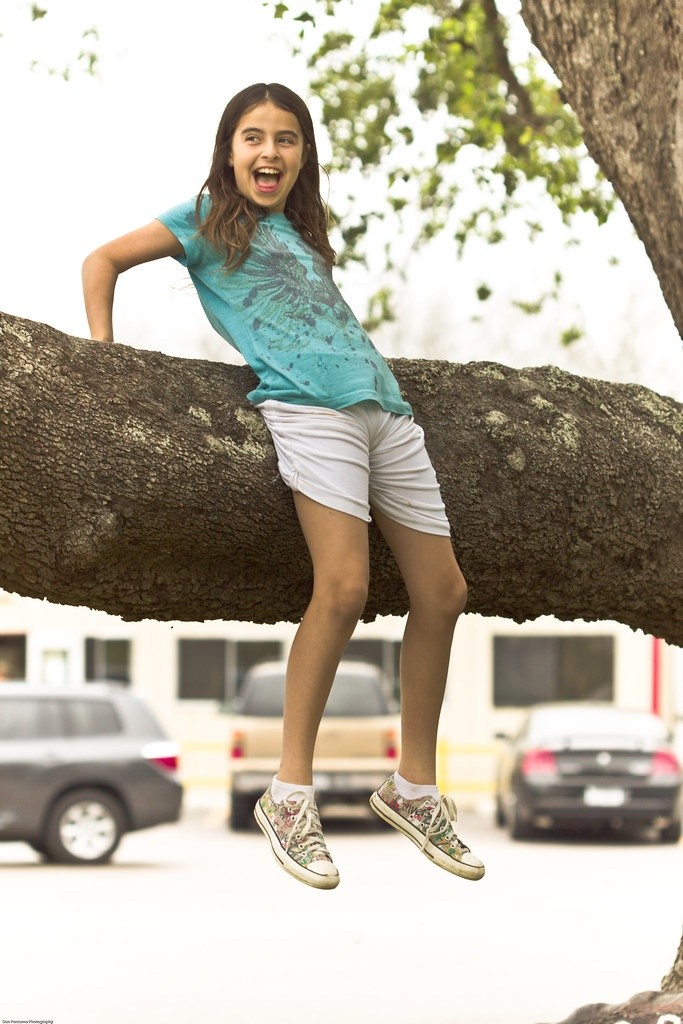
[253,785,340,891]
[368,773,486,881]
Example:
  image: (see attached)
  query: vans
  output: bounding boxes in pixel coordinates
[219,659,403,830]
[0,677,183,863]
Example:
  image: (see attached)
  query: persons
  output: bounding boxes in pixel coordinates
[81,83,485,890]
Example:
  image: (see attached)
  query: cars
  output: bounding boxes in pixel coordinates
[493,700,682,845]
